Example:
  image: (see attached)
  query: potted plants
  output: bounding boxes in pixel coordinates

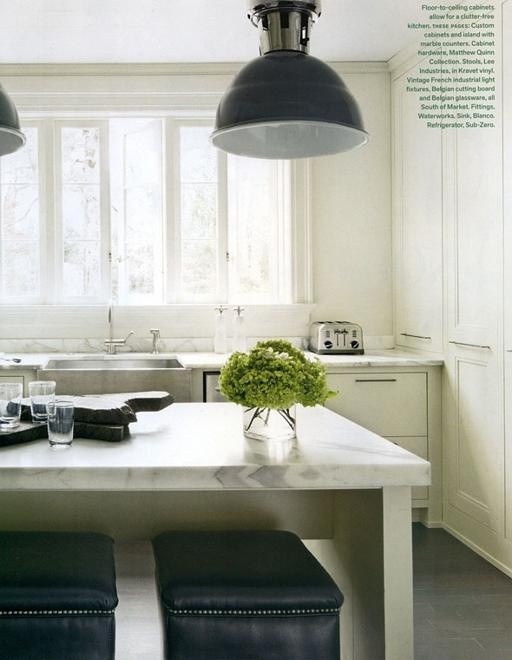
[218,334,336,466]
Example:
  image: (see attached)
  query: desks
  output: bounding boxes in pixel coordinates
[0,399,435,658]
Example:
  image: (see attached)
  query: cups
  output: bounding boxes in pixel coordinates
[0,383,22,428]
[27,379,57,425]
[47,401,74,448]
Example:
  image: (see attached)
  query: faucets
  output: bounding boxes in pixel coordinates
[105,331,134,354]
[150,328,162,355]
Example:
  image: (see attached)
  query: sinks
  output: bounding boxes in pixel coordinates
[39,352,187,373]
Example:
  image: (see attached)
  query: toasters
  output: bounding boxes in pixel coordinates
[310,321,364,356]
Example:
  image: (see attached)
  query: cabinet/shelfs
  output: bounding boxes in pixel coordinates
[319,367,432,514]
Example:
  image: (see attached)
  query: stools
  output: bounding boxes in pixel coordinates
[2,526,122,659]
[150,527,344,659]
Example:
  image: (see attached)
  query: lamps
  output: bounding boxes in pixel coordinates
[207,0,368,160]
